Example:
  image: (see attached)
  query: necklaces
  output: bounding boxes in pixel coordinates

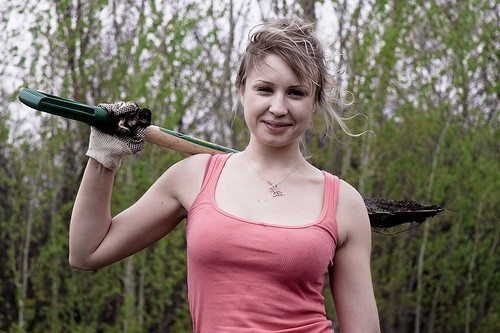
[244,149,303,196]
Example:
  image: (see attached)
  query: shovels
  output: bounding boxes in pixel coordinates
[18,86,447,236]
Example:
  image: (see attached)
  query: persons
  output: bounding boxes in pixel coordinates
[70,16,381,332]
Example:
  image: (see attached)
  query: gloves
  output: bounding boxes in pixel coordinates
[86,101,151,170]
